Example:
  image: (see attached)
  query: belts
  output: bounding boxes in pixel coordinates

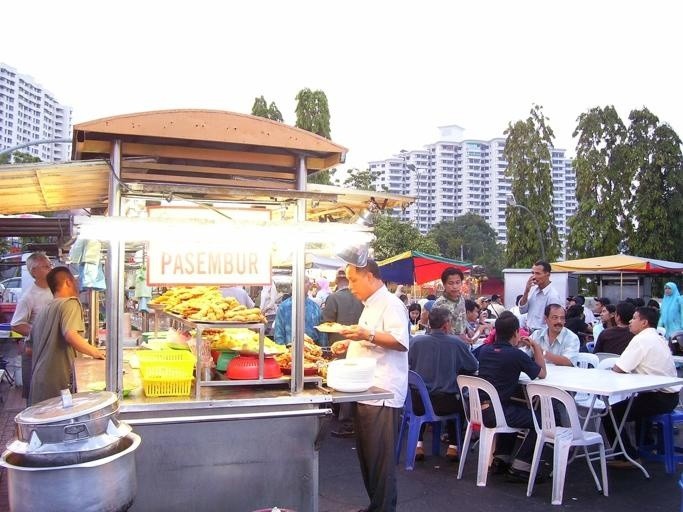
[481,400,492,403]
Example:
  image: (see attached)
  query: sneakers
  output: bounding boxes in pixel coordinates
[331,429,357,437]
[439,433,451,444]
[491,458,507,474]
[414,447,425,461]
[606,458,642,469]
[445,448,460,462]
[508,466,547,482]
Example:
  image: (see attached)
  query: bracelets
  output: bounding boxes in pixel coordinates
[366,331,375,343]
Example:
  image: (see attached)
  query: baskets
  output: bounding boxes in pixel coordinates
[210,349,281,379]
[136,349,198,396]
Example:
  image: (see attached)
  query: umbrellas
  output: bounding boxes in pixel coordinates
[375,249,473,305]
[549,252,683,299]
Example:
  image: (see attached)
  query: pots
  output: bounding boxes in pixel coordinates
[0,390,142,512]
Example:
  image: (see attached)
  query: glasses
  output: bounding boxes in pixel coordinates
[36,260,56,270]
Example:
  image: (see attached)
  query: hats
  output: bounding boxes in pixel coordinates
[336,266,347,278]
[566,296,584,305]
[627,297,644,307]
[594,297,609,305]
[482,295,500,301]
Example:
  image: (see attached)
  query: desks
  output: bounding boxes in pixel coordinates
[0,329,25,386]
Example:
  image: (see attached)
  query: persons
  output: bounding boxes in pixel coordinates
[382,260,682,482]
[25,266,105,405]
[329,257,410,511]
[217,267,360,366]
[656,280,683,340]
[9,253,77,400]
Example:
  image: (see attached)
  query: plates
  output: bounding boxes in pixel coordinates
[314,326,353,334]
[280,367,317,376]
[326,358,376,393]
